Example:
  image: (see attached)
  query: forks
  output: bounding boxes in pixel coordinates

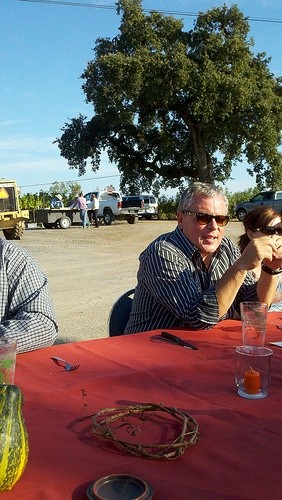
[50,356,80,371]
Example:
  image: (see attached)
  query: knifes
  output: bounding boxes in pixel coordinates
[162,332,199,350]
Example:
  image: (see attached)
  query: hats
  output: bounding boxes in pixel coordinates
[55,194,62,200]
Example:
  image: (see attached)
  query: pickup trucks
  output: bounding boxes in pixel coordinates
[235,191,282,221]
[70,191,144,225]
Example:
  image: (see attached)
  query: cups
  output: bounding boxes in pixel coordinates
[0,337,17,385]
[235,346,273,399]
[240,301,268,346]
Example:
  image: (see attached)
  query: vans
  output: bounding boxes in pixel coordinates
[122,195,159,221]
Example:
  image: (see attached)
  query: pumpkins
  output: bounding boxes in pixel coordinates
[0,385,28,491]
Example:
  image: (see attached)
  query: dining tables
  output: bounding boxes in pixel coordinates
[0,310,282,500]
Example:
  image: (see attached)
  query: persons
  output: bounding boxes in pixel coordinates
[125,182,282,335]
[88,194,99,228]
[236,207,282,312]
[0,230,58,354]
[50,194,64,209]
[71,192,90,229]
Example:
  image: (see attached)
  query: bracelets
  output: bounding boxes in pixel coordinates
[261,261,282,275]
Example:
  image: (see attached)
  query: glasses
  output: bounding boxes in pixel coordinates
[181,210,230,227]
[250,227,282,236]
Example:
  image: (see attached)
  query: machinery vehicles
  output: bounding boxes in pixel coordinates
[0,179,29,240]
[21,205,88,229]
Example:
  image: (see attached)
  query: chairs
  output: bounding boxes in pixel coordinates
[107,289,136,338]
